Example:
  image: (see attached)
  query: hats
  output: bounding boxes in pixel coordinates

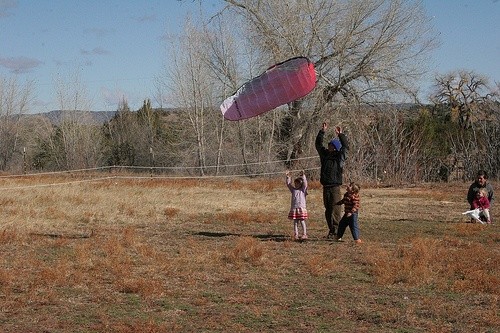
[328,140,341,151]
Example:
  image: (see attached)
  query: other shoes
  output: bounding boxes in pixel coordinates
[355,239,361,243]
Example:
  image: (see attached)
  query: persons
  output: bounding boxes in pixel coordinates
[316,122,351,241]
[332,183,365,245]
[467,169,495,224]
[286,168,310,240]
[471,188,492,224]
